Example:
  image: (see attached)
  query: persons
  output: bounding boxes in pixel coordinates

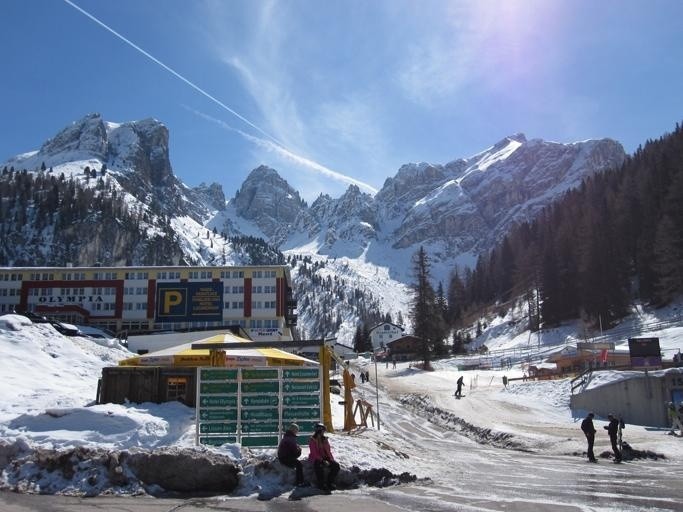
[360,371,365,383]
[308,422,340,490]
[365,370,369,382]
[392,358,396,370]
[277,420,307,484]
[453,375,466,397]
[665,401,683,437]
[350,373,355,382]
[602,412,623,463]
[581,412,598,462]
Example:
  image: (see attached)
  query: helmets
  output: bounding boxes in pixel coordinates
[313,423,327,431]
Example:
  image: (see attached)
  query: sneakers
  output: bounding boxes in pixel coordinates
[589,458,598,463]
[320,482,337,494]
[613,454,623,464]
